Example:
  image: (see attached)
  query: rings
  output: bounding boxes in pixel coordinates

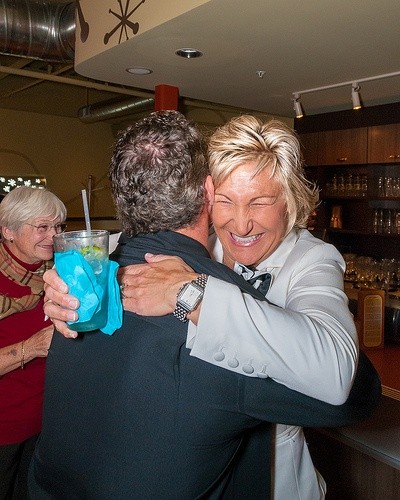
[42,299,52,309]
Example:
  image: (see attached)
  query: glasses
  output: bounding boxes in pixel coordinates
[24,221,68,236]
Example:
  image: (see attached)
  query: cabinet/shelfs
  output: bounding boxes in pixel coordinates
[299,134,319,167]
[312,190,400,239]
[317,126,369,166]
[367,124,400,162]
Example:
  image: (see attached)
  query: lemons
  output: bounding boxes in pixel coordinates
[81,247,104,260]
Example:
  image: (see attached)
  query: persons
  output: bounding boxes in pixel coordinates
[0,110,382,499]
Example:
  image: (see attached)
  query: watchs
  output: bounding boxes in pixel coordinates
[173,272,209,324]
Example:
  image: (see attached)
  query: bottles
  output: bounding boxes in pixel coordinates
[331,173,400,235]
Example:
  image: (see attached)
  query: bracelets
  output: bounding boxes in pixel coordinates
[21,338,24,369]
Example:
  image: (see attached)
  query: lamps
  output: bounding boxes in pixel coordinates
[292,94,304,117]
[349,82,363,110]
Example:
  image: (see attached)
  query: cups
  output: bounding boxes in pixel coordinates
[52,229,110,332]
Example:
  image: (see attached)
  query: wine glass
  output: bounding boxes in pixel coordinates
[343,254,400,291]
